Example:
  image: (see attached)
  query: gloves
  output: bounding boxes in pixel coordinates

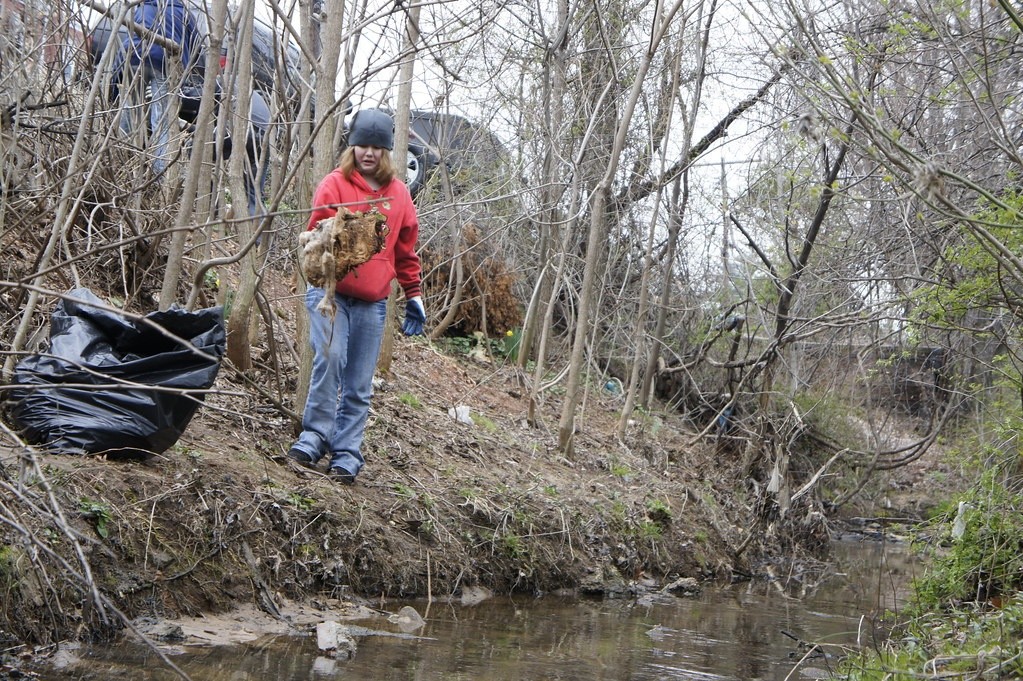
[401,296,426,336]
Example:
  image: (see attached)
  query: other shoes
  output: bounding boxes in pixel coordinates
[329,464,355,485]
[287,446,317,462]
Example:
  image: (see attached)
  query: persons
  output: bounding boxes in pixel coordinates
[275,106,431,482]
[141,67,277,250]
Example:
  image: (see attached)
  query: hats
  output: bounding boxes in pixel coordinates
[347,109,394,151]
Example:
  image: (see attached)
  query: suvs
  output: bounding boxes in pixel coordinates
[360,107,514,248]
[83,0,362,188]
